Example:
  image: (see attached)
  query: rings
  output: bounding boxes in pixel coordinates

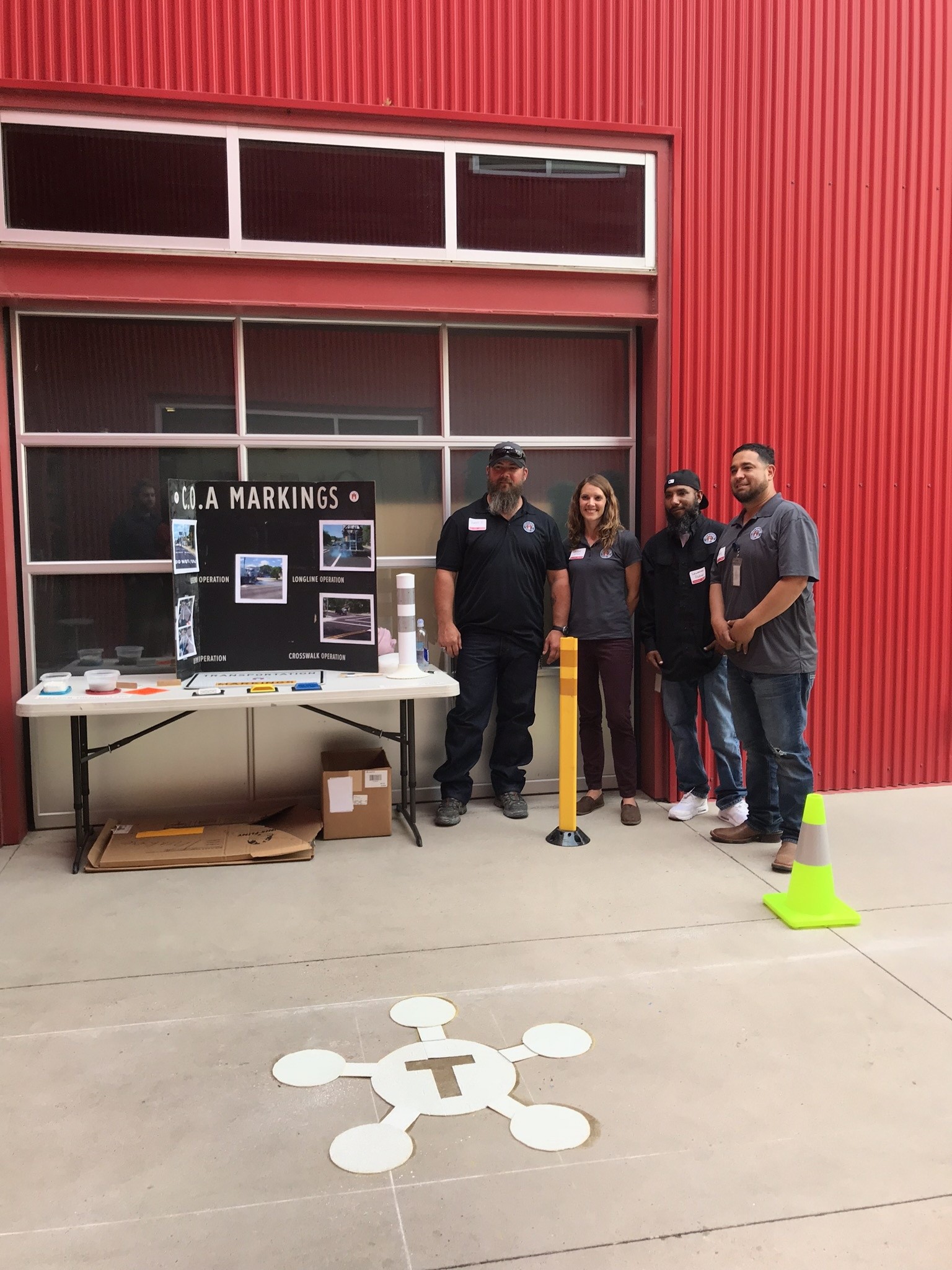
[441,646,446,653]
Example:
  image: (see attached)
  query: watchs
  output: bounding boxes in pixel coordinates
[552,625,569,636]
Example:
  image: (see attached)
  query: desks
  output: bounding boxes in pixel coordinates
[14,660,460,874]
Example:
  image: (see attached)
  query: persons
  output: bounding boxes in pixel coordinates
[709,444,820,873]
[636,469,749,828]
[562,473,643,826]
[434,442,572,826]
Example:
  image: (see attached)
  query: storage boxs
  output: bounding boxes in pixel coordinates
[320,748,392,839]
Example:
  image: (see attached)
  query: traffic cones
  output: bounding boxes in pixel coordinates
[762,793,861,929]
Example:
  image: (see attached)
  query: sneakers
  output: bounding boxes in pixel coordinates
[668,787,709,821]
[494,791,528,818]
[718,799,749,827]
[433,797,467,824]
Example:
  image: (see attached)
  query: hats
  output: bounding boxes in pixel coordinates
[664,469,709,510]
[490,442,526,468]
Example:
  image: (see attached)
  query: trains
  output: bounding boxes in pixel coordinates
[340,525,366,557]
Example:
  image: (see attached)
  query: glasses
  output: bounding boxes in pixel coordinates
[490,448,525,462]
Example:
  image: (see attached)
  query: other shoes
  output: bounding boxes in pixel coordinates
[620,799,641,825]
[576,792,604,815]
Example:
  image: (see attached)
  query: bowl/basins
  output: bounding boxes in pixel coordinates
[78,648,104,665]
[84,668,120,692]
[115,646,144,666]
[39,672,72,693]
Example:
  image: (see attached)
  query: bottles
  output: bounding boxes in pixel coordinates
[416,618,430,672]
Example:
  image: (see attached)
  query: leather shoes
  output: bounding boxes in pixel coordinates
[710,822,781,843]
[771,842,797,870]
